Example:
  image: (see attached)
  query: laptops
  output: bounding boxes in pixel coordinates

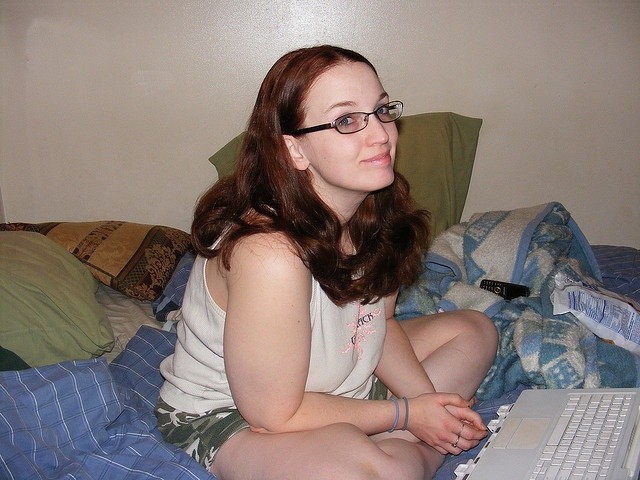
[464,385,640,480]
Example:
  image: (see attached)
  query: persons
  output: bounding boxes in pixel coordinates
[155,45,499,479]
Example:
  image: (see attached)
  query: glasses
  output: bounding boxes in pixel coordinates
[291,100,404,136]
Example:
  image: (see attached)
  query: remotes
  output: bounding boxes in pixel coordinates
[480,279,530,301]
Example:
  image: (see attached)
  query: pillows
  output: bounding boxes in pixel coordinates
[0,218,193,301]
[208,112,482,244]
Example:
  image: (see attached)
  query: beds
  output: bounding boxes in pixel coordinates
[0,232,640,479]
